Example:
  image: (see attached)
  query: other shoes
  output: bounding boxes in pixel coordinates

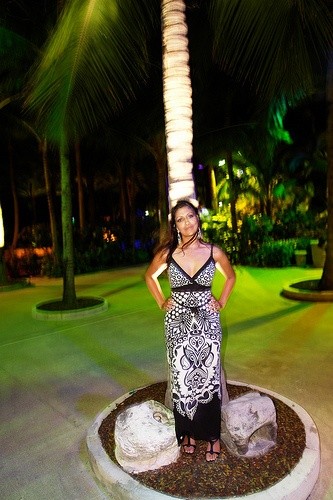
[205,437,221,462]
[183,436,196,455]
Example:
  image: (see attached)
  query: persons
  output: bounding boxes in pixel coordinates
[144,199,237,462]
